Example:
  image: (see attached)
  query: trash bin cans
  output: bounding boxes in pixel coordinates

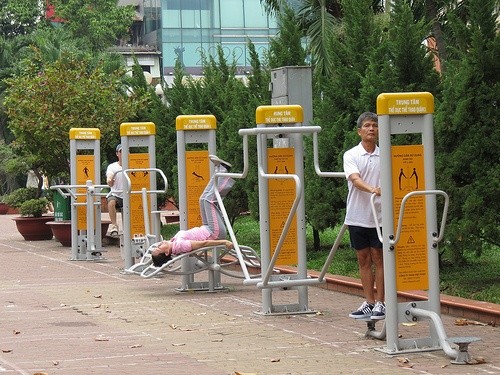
[50,190,71,221]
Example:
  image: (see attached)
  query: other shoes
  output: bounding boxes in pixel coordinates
[111,224,119,236]
[208,155,233,172]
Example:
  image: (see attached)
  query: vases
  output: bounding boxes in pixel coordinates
[162,213,180,223]
[46,220,112,247]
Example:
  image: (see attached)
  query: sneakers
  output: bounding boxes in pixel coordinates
[349,301,374,319]
[371,301,385,320]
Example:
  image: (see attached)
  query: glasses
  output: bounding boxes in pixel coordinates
[115,149,121,153]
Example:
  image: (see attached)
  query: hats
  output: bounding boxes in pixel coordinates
[116,144,122,152]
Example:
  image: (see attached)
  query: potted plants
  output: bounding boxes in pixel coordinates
[0,186,57,241]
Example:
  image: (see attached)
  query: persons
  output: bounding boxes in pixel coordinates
[152,154,237,267]
[105,144,130,238]
[20,159,57,216]
[341,111,388,320]
